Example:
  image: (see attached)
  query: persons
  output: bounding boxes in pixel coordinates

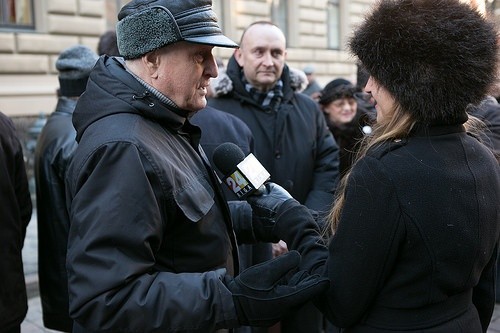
[36,46,99,332]
[244,0,500,333]
[0,113,34,333]
[97,30,123,57]
[64,0,332,333]
[188,22,499,333]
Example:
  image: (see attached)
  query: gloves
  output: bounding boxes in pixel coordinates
[245,180,301,236]
[255,208,328,239]
[227,250,332,328]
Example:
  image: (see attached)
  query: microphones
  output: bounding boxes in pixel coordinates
[213,142,272,200]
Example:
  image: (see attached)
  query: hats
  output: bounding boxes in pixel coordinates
[342,0,500,128]
[318,79,362,105]
[115,0,240,57]
[96,30,124,59]
[56,44,98,97]
